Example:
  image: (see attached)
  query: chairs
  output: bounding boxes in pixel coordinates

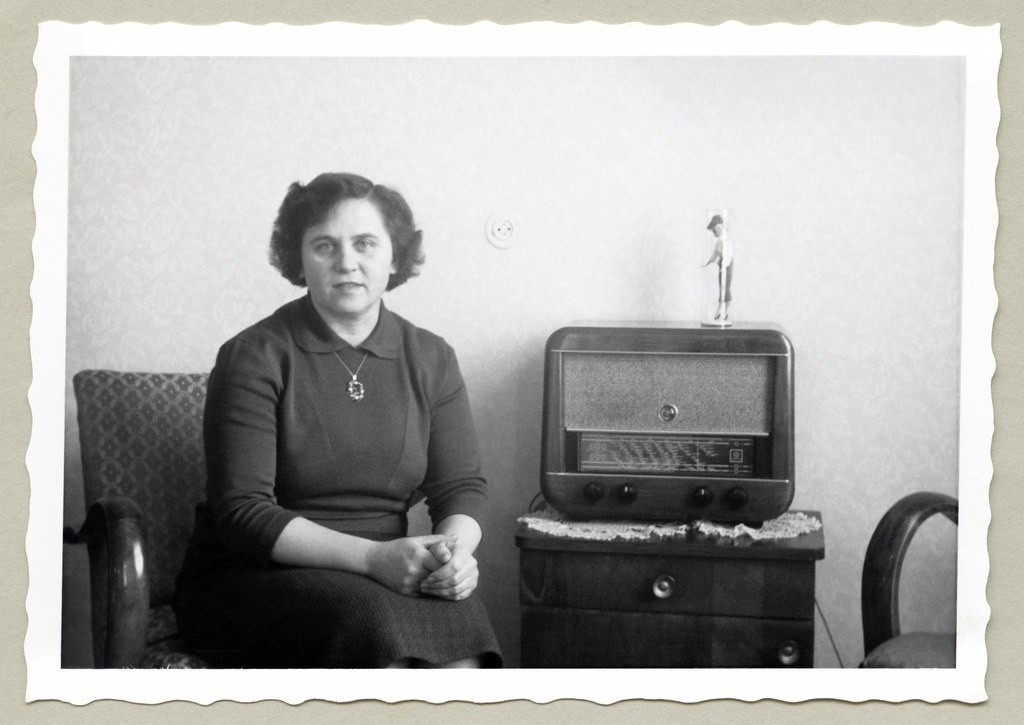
[858,492,959,668]
[62,370,214,669]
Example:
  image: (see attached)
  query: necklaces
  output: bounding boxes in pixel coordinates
[333,350,369,402]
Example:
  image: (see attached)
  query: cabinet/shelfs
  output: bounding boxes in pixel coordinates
[513,510,825,667]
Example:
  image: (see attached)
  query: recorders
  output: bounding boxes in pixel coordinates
[541,323,795,524]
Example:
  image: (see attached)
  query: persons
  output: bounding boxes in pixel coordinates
[201,172,504,669]
[703,215,735,327]
